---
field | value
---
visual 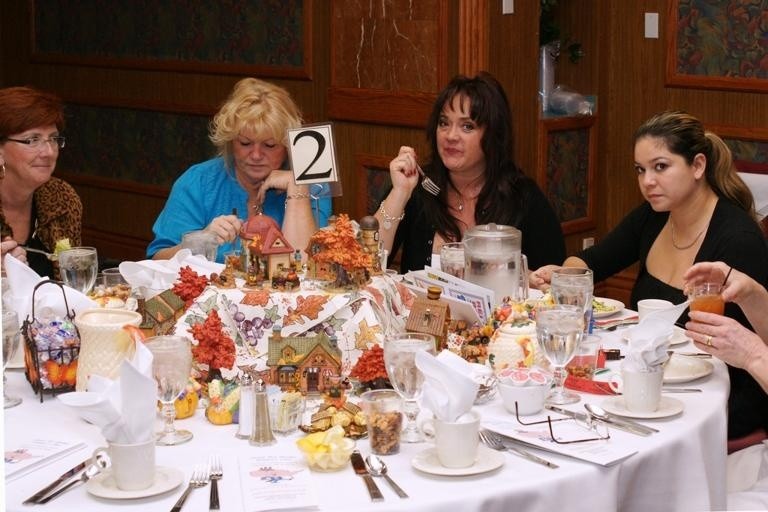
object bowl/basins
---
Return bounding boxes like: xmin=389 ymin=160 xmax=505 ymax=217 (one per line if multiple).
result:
xmin=299 ymin=438 xmax=354 ymax=473
xmin=497 ymin=382 xmax=550 ymax=415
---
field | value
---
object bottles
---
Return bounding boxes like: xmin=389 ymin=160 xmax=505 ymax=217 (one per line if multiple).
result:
xmin=235 ymin=374 xmax=254 ymax=440
xmin=251 ymin=380 xmax=278 ymax=444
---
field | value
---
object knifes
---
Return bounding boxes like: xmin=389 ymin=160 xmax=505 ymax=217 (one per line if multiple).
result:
xmin=22 ymin=458 xmax=95 ymax=505
xmin=352 ymin=450 xmax=386 ymax=503
xmin=542 ymin=402 xmax=646 ymax=442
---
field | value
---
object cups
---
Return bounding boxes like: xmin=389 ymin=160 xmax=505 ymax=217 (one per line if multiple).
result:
xmin=92 ymin=435 xmax=156 ymax=490
xmin=539 ymin=46 xmax=553 ymax=112
xmin=104 ymin=268 xmax=126 ymax=287
xmin=86 ymin=466 xmax=182 ymax=500
xmin=609 ymin=366 xmax=663 ymax=412
xmin=415 ymin=413 xmax=480 ymax=467
xmin=637 ymin=299 xmax=674 ymax=338
xmin=549 ymin=87 xmax=584 ymax=119
xmin=183 ymin=231 xmax=217 ymax=263
xmin=361 ymin=389 xmax=405 ymax=456
xmin=686 ymin=282 xmax=726 ymax=333
xmin=565 ymin=334 xmax=602 ymax=382
xmin=439 ymin=243 xmax=466 ymax=281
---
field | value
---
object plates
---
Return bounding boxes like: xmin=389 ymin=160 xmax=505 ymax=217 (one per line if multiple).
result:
xmin=609 ymin=352 xmax=712 ymax=382
xmin=411 ymin=445 xmax=508 ymax=477
xmin=594 ymin=296 xmax=626 ymax=317
xmin=620 ymin=325 xmax=690 ymax=347
xmin=603 ymin=396 xmax=683 ymax=420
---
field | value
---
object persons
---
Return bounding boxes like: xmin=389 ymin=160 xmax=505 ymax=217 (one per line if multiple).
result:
xmin=683 ymin=259 xmax=768 ymax=511
xmin=146 ymin=78 xmax=333 ymax=264
xmin=527 ymin=111 xmax=768 ymax=440
xmin=372 ymin=75 xmax=566 ymax=289
xmin=0 ymin=87 xmax=83 ymax=282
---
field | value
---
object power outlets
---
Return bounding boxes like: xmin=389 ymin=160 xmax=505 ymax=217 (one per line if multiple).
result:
xmin=583 ymin=237 xmax=595 ymax=252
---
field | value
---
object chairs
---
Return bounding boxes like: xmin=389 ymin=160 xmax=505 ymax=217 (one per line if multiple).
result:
xmin=729 ymin=169 xmax=767 ymax=460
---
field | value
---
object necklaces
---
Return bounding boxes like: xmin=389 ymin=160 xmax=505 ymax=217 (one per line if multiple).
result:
xmin=248 ymin=199 xmax=265 ymax=216
xmin=669 ymin=213 xmax=714 ymax=250
xmin=445 ymin=180 xmax=481 ymax=212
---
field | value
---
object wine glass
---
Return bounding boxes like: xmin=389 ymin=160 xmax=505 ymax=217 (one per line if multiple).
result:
xmin=384 ymin=333 xmax=436 ymax=444
xmin=551 ymin=268 xmax=594 ymax=345
xmin=2 ymin=310 xmax=24 ymax=409
xmin=537 ymin=304 xmax=584 ymax=404
xmin=141 ymin=336 xmax=194 ymax=447
xmin=58 ymin=247 xmax=98 ymax=297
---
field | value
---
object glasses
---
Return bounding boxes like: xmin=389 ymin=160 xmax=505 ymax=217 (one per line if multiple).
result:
xmin=6 ymin=136 xmax=66 ymax=150
xmin=515 ymin=401 xmax=610 ymax=444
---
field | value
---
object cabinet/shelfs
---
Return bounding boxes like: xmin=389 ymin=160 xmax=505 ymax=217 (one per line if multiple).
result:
xmin=1 ymin=2 xmax=318 ymax=271
xmin=316 ymin=0 xmax=609 ymax=262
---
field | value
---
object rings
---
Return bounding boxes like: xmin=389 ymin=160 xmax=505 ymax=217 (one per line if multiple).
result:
xmin=260 ymin=179 xmax=265 ymax=186
xmin=706 ymin=336 xmax=712 ymax=346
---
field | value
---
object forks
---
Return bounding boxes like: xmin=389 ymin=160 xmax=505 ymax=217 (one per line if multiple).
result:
xmin=596 ymin=323 xmax=640 ymax=334
xmin=414 ymin=163 xmax=440 ymax=196
xmin=170 ymin=470 xmax=208 ymax=512
xmin=17 ymin=246 xmax=59 ymax=262
xmin=209 ymin=456 xmax=224 ymax=510
xmin=478 ymin=433 xmax=560 ymax=471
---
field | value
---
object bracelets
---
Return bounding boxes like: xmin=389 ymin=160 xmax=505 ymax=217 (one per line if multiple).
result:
xmin=719 ymin=267 xmax=735 ymax=295
xmin=379 ymin=199 xmax=405 ymax=229
xmin=284 ymin=193 xmax=311 ymax=212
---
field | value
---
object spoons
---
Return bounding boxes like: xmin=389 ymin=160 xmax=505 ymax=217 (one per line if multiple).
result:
xmin=39 ymin=462 xmax=104 ymax=505
xmin=584 ymin=404 xmax=661 ymax=436
xmin=366 ymin=456 xmax=410 ymax=502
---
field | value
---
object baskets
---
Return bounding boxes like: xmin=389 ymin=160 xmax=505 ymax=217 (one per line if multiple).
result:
xmin=20 ymin=280 xmax=80 ymax=402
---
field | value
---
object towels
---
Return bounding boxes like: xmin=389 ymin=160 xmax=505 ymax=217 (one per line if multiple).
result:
xmin=414 ymin=347 xmax=495 ymax=423
xmin=118 ymin=248 xmax=227 ymax=301
xmin=619 ymin=295 xmax=695 ymax=370
xmin=3 ymin=251 xmax=91 ymax=318
xmin=50 ymin=337 xmax=165 ymax=447
